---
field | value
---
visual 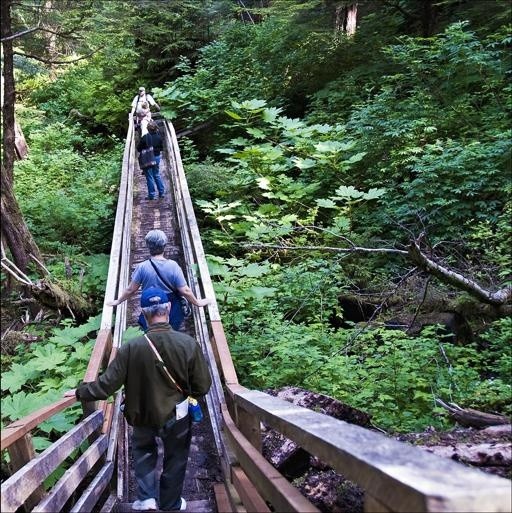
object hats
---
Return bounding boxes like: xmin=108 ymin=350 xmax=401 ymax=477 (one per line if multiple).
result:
xmin=141 ymin=287 xmax=169 ymax=307
xmin=139 ymin=86 xmax=146 ymax=90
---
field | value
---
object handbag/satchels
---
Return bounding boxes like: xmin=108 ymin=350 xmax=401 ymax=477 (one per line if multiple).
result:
xmin=187 ymin=396 xmax=204 ymax=422
xmin=179 ymin=295 xmax=192 ymax=316
xmin=138 ymin=144 xmax=157 ymax=169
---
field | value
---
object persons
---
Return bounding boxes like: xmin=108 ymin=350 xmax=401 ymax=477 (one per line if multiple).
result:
xmin=136 ymin=101 xmax=151 ymax=141
xmin=105 ymin=228 xmax=213 ymax=331
xmin=137 ymin=121 xmax=166 ymax=199
xmin=64 ymin=283 xmax=212 ymax=513
xmin=140 ymin=108 xmax=158 ymax=174
xmin=126 ymin=86 xmax=161 ymax=118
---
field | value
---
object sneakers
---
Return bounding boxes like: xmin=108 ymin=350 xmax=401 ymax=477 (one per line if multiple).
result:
xmin=180 ymin=495 xmax=187 ymax=511
xmin=145 ymin=195 xmax=155 ymax=199
xmin=132 ymin=497 xmax=158 ymax=511
xmin=159 ymin=193 xmax=165 ymax=197
